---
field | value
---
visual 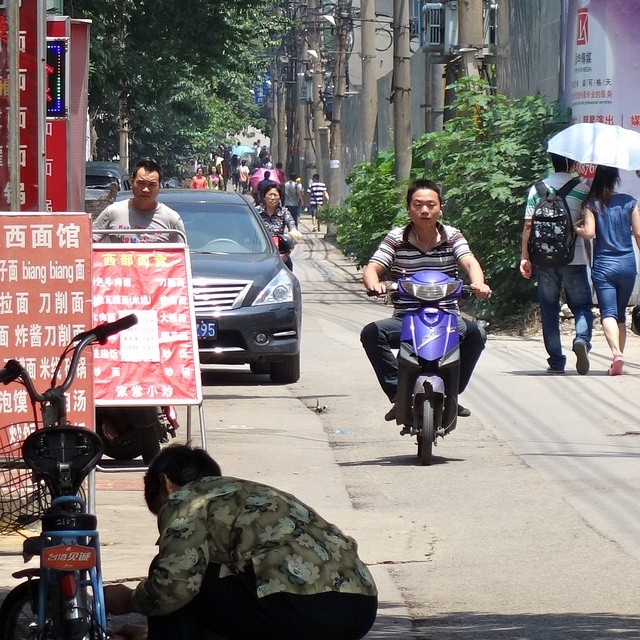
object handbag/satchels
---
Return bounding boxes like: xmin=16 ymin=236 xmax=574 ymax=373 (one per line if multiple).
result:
xmin=631 ymin=291 xmax=640 ymax=336
xmin=218 ymin=173 xmax=227 ymax=192
xmin=295 ymin=183 xmax=305 ymax=206
xmin=311 ymin=186 xmax=324 ymax=214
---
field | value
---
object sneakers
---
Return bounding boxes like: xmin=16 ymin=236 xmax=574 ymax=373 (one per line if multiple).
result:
xmin=573 ymin=338 xmax=591 ymax=375
xmin=313 ymin=227 xmax=318 ymax=233
xmin=547 ymin=368 xmax=565 ymax=374
xmin=319 ymin=228 xmax=322 ymax=232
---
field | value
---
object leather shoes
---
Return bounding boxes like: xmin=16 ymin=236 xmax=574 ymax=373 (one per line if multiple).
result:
xmin=458 ymin=404 xmax=472 ymax=417
xmin=386 ymin=402 xmax=397 ymax=423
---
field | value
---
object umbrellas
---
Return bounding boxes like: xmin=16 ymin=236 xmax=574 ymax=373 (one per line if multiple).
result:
xmin=544 ymin=123 xmax=640 ymax=171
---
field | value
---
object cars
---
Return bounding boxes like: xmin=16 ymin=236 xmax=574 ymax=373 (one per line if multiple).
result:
xmin=115 ymin=190 xmax=300 ymax=384
xmin=86 ymin=162 xmax=130 ymax=191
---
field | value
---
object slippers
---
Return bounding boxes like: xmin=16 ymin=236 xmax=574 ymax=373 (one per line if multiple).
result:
xmin=609 ymin=357 xmax=626 ymax=376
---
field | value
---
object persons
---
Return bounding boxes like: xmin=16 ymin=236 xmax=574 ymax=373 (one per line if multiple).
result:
xmin=103 ymin=442 xmax=378 ymax=640
xmin=305 ymin=173 xmax=329 ymax=232
xmin=254 ymin=184 xmax=297 ymax=236
xmin=359 ymin=178 xmax=490 ymax=421
xmin=92 ymin=161 xmax=187 ymax=244
xmin=520 ymin=154 xmax=591 ymax=375
xmin=284 ymin=174 xmax=304 ymax=229
xmin=572 ymin=165 xmax=640 ymax=375
xmin=189 ymin=140 xmax=286 ymax=190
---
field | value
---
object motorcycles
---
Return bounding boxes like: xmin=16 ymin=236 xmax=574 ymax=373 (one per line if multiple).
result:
xmin=95 ymin=406 xmax=179 ymax=466
xmin=0 ymin=314 xmax=139 ymax=640
xmin=367 ymin=271 xmax=491 ymax=462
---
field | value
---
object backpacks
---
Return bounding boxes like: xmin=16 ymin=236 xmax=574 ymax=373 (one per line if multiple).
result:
xmin=528 ymin=177 xmax=583 ymax=269
